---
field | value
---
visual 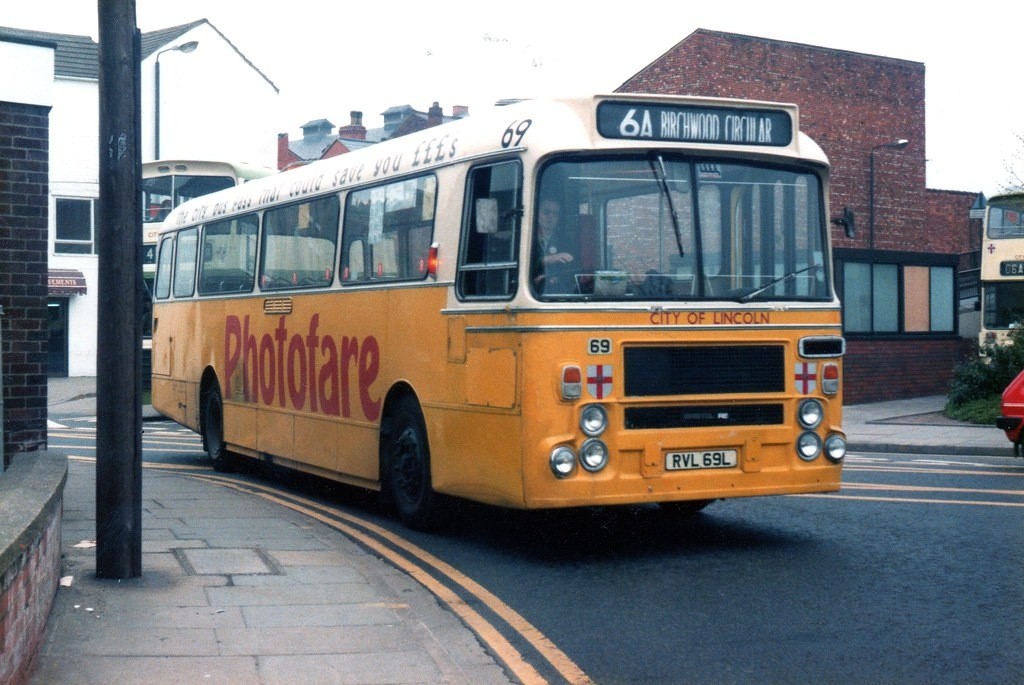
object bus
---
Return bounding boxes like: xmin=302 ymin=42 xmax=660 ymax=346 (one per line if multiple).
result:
xmin=141 ymin=160 xmax=282 ymax=390
xmin=150 ymin=92 xmax=849 ymax=528
xmin=974 ymin=191 xmax=1024 ymax=364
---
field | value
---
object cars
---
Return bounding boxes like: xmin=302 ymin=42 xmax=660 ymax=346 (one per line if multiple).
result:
xmin=994 ymin=368 xmax=1024 ymax=458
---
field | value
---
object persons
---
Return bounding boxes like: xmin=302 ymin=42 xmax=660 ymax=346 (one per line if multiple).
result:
xmin=150 ymin=199 xmax=171 ymax=222
xmin=521 ymin=198 xmax=579 ymax=296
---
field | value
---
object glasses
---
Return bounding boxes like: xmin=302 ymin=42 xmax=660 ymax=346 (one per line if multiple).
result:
xmin=541 ymin=208 xmax=559 ymax=216
